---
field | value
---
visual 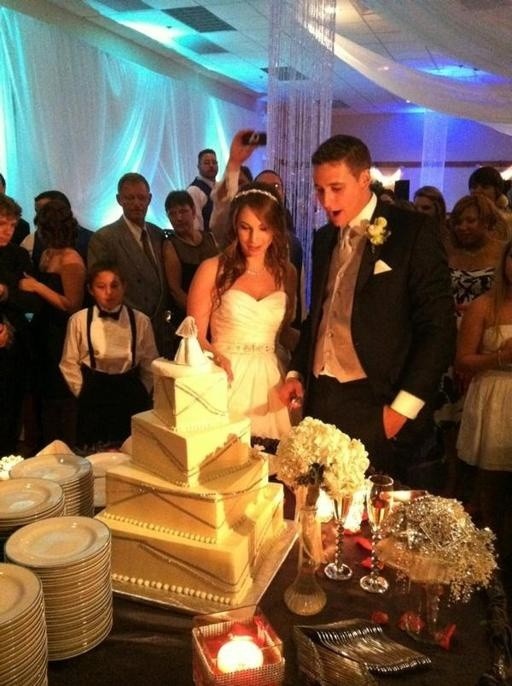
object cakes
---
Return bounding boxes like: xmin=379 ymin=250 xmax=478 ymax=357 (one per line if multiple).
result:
xmin=92 ymin=315 xmax=286 ymax=606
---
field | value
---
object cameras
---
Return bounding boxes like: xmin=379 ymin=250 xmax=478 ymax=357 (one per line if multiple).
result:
xmin=241 ymin=130 xmax=268 ymax=146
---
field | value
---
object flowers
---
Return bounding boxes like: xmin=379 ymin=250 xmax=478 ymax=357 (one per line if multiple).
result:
xmin=275 ymin=415 xmax=371 ymax=563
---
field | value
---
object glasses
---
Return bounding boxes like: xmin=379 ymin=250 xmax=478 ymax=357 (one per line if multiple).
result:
xmin=271 ymin=183 xmax=283 ymax=190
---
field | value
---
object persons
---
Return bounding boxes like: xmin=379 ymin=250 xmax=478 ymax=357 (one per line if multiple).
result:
xmin=0 ymin=127 xmax=510 ymax=534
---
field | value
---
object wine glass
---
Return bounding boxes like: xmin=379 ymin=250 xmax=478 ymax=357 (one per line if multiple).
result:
xmin=324 ymin=492 xmax=352 ymax=581
xmin=361 ymin=474 xmax=393 ymax=594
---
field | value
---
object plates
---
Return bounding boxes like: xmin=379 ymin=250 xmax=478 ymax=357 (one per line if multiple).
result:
xmin=1 ymin=452 xmax=134 ymax=685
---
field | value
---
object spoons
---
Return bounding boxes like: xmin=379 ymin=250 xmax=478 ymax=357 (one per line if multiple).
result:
xmin=309 ymin=619 xmax=433 ymax=677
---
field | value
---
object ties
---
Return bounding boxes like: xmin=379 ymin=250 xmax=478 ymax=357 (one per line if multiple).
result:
xmin=140 ymin=229 xmax=160 ymax=277
xmin=336 ymin=226 xmax=355 ymax=267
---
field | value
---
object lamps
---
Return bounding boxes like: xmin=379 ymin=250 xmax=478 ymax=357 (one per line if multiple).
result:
xmin=191 ymin=604 xmax=286 ymax=685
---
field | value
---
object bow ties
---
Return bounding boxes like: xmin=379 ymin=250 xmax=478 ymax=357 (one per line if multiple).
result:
xmin=99 ymin=310 xmax=120 ymax=321
